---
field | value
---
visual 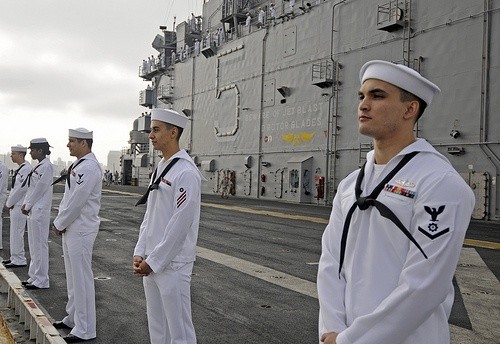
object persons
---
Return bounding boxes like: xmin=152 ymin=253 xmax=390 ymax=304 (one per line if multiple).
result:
xmin=142 ymin=0 xmax=312 ymax=75
xmin=134 ymin=107 xmax=199 ymax=343
xmin=104 ymin=170 xmax=123 ymax=191
xmin=49 ymin=127 xmax=103 ymax=343
xmin=5 ymin=144 xmax=27 ymax=268
xmin=314 ymin=58 xmax=475 ymax=342
xmin=142 ymin=84 xmax=152 ymax=117
xmin=21 ymin=139 xmax=49 ymax=291
xmin=219 ymin=175 xmax=232 ymax=200
xmin=0 ymin=161 xmax=10 ymax=251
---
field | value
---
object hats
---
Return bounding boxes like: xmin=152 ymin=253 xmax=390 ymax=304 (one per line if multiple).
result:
xmin=11 ymin=145 xmax=28 ymax=152
xmin=151 ymin=108 xmax=188 ymax=129
xmin=359 ymin=60 xmax=441 ymax=107
xmin=28 ymin=138 xmax=51 ymax=148
xmin=69 ymin=128 xmax=93 ymax=139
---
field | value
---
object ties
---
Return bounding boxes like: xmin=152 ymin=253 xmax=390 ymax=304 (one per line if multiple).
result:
xmin=11 ymin=163 xmax=25 ymax=188
xmin=134 ymin=158 xmax=180 ymax=206
xmin=338 ymin=152 xmax=427 ymax=279
xmin=21 ymin=163 xmax=41 ymax=188
xmin=51 ymin=159 xmax=86 ymax=189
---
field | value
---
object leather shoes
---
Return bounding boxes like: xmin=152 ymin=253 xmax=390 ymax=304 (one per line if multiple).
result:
xmin=3 ymin=259 xmax=96 ymax=343
